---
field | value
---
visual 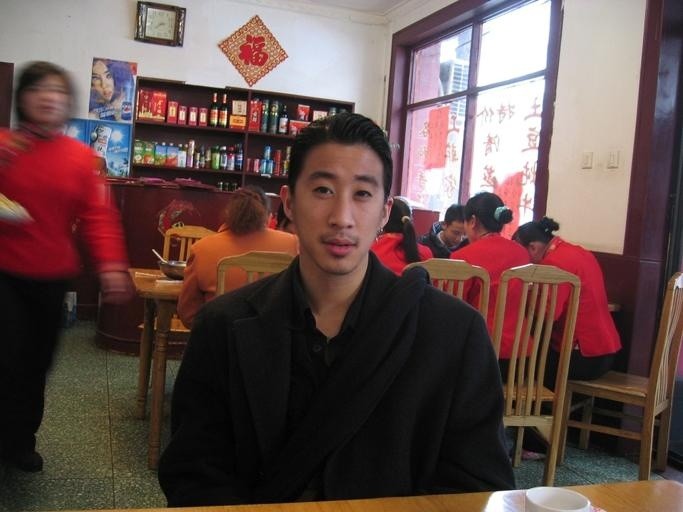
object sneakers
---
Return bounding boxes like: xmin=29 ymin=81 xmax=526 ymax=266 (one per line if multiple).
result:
xmin=509 ymin=444 xmax=548 ymax=462
xmin=1 ymin=443 xmax=44 ymax=473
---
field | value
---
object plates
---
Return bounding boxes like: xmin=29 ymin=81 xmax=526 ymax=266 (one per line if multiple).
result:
xmin=484 ymin=489 xmax=607 ymax=511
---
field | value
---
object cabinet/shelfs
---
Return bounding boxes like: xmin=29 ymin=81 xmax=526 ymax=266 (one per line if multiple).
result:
xmin=129 ymin=75 xmax=356 ymax=191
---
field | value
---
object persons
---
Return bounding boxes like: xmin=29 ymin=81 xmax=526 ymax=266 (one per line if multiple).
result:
xmin=0 ymin=62 xmax=136 ymax=472
xmin=90 ymin=58 xmax=134 ymax=123
xmin=157 ymin=111 xmax=518 ymax=506
xmin=178 ymin=187 xmax=298 ymax=329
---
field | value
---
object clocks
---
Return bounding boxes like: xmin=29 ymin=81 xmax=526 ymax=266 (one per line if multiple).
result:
xmin=134 ymin=0 xmax=186 ymax=47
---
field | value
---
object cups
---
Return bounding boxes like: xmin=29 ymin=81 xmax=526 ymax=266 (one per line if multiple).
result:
xmin=216 ymin=181 xmax=238 ymax=192
xmin=524 ymin=485 xmax=593 ymax=511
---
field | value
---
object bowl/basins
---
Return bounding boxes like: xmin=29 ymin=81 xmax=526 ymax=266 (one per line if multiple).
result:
xmin=157 ymin=261 xmax=185 ymax=280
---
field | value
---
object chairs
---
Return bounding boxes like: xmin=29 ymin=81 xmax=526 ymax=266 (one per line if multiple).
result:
xmin=552 ymin=270 xmax=683 ymax=481
xmin=160 ymin=226 xmax=217 ymax=261
xmin=400 ymin=258 xmax=490 ymax=325
xmin=213 ymin=250 xmax=295 ymax=297
xmin=490 ymin=262 xmax=582 ymax=487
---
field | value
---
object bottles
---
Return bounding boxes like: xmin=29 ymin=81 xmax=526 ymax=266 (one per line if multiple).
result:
xmin=219 ymin=94 xmax=230 ymax=126
xmin=209 ymin=93 xmax=218 ymax=127
xmin=328 ymin=106 xmax=346 ymax=117
xmin=277 ymin=104 xmax=289 ymax=135
xmin=177 ymin=139 xmax=242 ymax=171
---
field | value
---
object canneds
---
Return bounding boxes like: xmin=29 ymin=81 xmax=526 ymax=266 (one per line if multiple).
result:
xmin=246 ymin=144 xmax=292 ymax=176
xmin=186 ymin=140 xmax=200 ymax=169
xmin=121 ymin=102 xmax=131 ymax=120
